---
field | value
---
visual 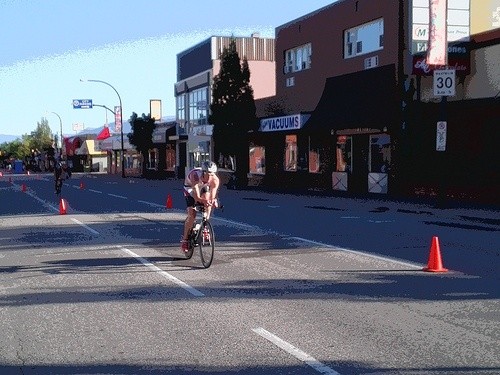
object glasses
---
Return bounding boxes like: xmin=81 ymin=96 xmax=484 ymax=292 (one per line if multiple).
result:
xmin=204 ymin=172 xmax=215 ymax=176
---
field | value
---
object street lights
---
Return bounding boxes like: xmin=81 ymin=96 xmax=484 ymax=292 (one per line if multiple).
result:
xmin=80 ymin=78 xmax=126 ymax=179
xmin=44 ymin=110 xmax=63 ymax=160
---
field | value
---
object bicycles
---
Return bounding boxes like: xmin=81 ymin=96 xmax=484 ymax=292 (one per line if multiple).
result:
xmin=53 ymin=177 xmax=63 ymax=197
xmin=179 ymin=201 xmax=224 ymax=268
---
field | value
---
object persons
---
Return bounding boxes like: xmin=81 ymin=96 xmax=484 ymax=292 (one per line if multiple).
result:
xmin=181 ymin=160 xmax=220 ymax=253
xmin=54 ymin=163 xmax=65 ymax=194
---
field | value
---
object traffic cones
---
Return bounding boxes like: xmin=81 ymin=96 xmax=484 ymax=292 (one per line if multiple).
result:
xmin=165 ymin=193 xmax=173 ymax=209
xmin=423 ymin=237 xmax=448 ymax=272
xmin=80 ymin=182 xmax=85 ymax=191
xmin=60 ymin=198 xmax=67 ymax=214
xmin=22 ymin=183 xmax=26 ymax=191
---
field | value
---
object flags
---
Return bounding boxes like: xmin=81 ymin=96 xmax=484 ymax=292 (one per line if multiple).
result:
xmin=95 ymin=127 xmax=110 ymax=140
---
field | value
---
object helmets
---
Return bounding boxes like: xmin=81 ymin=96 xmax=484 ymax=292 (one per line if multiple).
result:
xmin=202 ymin=161 xmax=217 ymax=172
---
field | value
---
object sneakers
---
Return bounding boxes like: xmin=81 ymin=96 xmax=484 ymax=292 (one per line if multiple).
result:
xmin=204 ymin=230 xmax=209 ymax=240
xmin=182 ymin=239 xmax=189 ymax=253
